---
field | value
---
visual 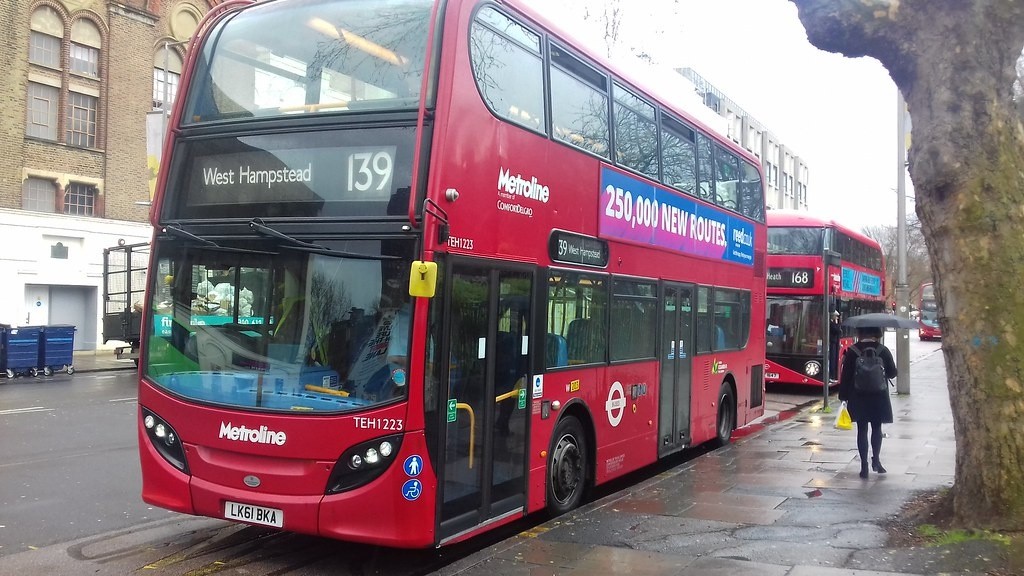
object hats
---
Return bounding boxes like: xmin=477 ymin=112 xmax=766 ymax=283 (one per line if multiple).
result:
xmin=830 ymin=310 xmax=840 ymax=316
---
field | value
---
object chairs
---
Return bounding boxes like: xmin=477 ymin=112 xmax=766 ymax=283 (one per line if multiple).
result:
xmin=325 ymin=313 xmax=391 ymax=400
xmin=470 ymin=310 xmax=729 ymax=385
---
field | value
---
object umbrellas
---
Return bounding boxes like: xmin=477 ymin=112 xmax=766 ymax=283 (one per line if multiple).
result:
xmin=842 ymin=313 xmax=923 ymax=346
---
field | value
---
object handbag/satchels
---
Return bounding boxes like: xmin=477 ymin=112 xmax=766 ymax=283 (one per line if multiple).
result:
xmin=833 ymin=400 xmax=853 ymax=430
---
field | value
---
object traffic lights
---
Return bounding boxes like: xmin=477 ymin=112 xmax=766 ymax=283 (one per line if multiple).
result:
xmin=892 ymin=302 xmax=896 ymax=309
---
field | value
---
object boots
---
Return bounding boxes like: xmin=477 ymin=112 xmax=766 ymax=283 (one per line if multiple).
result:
xmin=871 ymin=456 xmax=886 ymax=474
xmin=859 ymin=462 xmax=868 ymax=480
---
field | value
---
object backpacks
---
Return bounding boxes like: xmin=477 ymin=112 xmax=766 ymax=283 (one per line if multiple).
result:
xmin=847 ymin=344 xmax=889 ymax=399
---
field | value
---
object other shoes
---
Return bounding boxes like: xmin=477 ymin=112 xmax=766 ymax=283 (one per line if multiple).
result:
xmin=828 ymin=381 xmax=832 ymax=385
xmin=830 ymin=379 xmax=838 ymax=383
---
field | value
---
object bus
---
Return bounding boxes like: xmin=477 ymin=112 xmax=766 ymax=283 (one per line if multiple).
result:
xmin=919 ymin=283 xmax=942 ymax=342
xmin=764 ymin=215 xmax=885 ymax=390
xmin=137 ymin=1 xmax=767 ymax=550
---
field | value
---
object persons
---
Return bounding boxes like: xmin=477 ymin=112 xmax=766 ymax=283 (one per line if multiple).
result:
xmin=279 ymin=254 xmax=332 ymax=321
xmin=387 ymin=278 xmax=437 ymax=469
xmin=830 ymin=310 xmax=841 ymax=383
xmin=837 ymin=327 xmax=898 ymax=479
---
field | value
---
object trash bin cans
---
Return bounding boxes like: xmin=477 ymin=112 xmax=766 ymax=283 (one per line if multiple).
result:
xmin=0 ymin=324 xmax=44 ymax=379
xmin=38 ymin=324 xmax=77 ymax=377
